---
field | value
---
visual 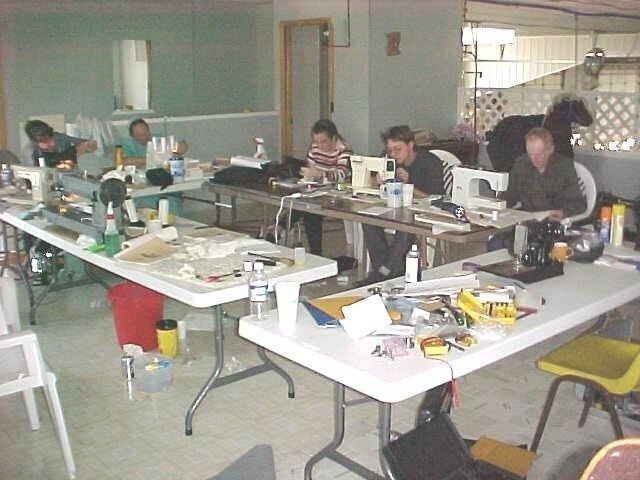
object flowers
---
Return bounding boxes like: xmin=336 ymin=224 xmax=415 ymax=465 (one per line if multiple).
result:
xmin=447 ymin=122 xmax=483 ymax=143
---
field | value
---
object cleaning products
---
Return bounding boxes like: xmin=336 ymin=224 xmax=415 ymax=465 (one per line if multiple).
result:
xmin=103 ymin=200 xmax=121 ymax=257
xmin=251 ymin=137 xmax=268 ymax=161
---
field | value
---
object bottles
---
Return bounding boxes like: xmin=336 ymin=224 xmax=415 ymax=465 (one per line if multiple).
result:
xmin=403 ymin=244 xmax=424 ymax=284
xmin=0 ymin=164 xmax=27 ymax=194
xmin=102 ymin=201 xmax=120 ymax=255
xmin=293 ymin=241 xmax=306 ymax=265
xmin=598 ymin=202 xmax=627 ymax=251
xmin=168 ymin=150 xmax=185 ymax=181
xmin=156 ymin=319 xmax=178 ymax=358
xmin=247 ymin=261 xmax=270 ymax=323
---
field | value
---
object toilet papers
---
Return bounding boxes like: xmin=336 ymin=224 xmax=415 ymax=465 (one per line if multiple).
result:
xmin=121 ymin=226 xmax=178 ymax=250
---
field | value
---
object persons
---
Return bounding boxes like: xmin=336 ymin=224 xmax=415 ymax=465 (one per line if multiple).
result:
xmin=23 ymin=120 xmax=98 ymax=254
xmin=266 ymin=119 xmax=353 ymax=256
xmin=120 ymin=119 xmax=188 ymax=217
xmin=351 ymin=126 xmax=445 ymax=288
xmin=487 ymin=128 xmax=587 ymax=251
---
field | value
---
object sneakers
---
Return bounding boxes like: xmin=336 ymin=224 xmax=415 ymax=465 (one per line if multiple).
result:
xmin=263 ymin=222 xmax=284 ymax=243
xmin=351 ymin=275 xmax=387 ymax=289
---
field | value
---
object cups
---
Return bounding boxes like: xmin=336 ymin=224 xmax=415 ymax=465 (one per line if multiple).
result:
xmin=379 ymin=178 xmax=415 ymax=211
xmin=120 ymin=351 xmax=134 ymax=380
xmin=514 ymin=284 xmax=544 ymax=314
xmin=515 ymin=240 xmax=575 ymax=268
xmin=273 ymin=282 xmax=302 ymax=324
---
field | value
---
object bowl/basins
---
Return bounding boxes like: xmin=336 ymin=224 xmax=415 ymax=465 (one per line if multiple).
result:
xmin=132 ymin=354 xmax=176 ymax=394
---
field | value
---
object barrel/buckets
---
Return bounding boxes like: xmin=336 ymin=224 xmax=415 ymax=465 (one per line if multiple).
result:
xmin=104 ymin=284 xmax=166 ymax=352
